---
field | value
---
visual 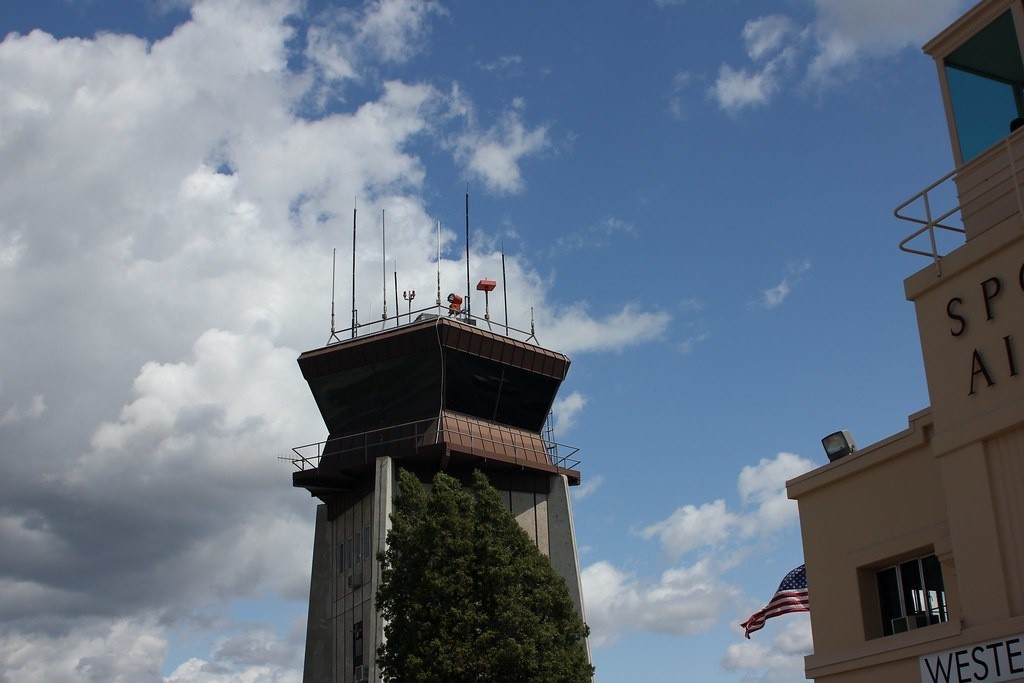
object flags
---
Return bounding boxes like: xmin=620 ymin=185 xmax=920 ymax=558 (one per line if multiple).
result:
xmin=739 ymin=563 xmax=812 ymax=640
